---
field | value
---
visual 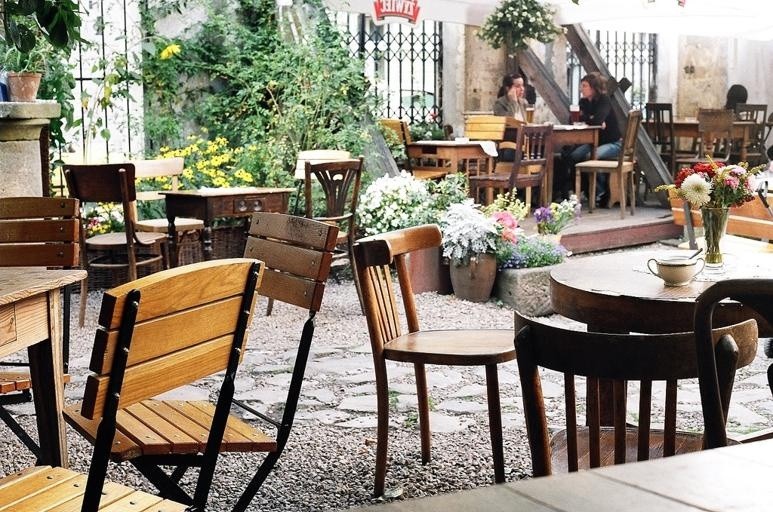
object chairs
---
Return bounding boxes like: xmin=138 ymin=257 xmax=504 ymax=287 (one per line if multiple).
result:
xmin=352 ymin=224 xmax=517 ymax=497
xmin=512 ymin=309 xmax=758 ymax=477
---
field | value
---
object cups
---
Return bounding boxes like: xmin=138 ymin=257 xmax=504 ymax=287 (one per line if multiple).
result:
xmin=647 ymin=258 xmax=705 ymax=287
xmin=570 ymin=105 xmax=581 ymax=125
xmin=525 ymin=103 xmax=536 ymax=123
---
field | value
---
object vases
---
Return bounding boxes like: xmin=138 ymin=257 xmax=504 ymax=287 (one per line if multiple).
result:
xmin=395 ymin=246 xmax=449 ymax=295
xmin=699 ymin=209 xmax=728 ymax=272
xmin=446 ymin=251 xmax=499 ymax=303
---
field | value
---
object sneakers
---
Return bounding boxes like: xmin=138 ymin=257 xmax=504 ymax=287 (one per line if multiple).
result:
xmin=598 ymin=191 xmax=610 ymax=209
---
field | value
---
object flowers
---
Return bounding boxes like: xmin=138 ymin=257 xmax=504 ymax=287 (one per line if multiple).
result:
xmin=654 ymin=152 xmax=766 ymax=263
xmin=475 ymin=1 xmax=568 ymax=56
xmin=357 ymin=175 xmax=582 ymax=265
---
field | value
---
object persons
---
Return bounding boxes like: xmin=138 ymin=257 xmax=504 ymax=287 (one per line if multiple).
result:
xmin=492 ymin=71 xmax=529 ymax=163
xmin=559 ymin=72 xmax=622 ymax=204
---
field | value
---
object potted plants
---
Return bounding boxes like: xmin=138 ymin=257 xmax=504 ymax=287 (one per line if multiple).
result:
xmin=0 ymin=15 xmax=54 ymax=101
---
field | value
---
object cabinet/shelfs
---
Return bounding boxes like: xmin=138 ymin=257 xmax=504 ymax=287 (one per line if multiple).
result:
xmin=694 ymin=280 xmax=773 ymax=447
